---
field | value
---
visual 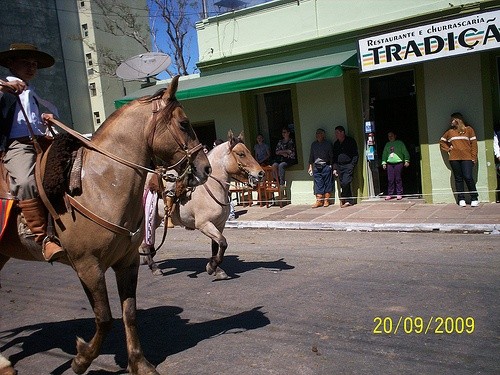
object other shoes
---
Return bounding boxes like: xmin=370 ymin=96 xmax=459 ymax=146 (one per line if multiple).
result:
xmin=385 ymin=197 xmax=391 ymax=200
xmin=396 ymin=195 xmax=402 ymax=199
xmin=276 ymin=185 xmax=283 ymax=188
xmin=341 ymin=202 xmax=352 ymax=207
xmin=271 ymin=184 xmax=275 ymax=188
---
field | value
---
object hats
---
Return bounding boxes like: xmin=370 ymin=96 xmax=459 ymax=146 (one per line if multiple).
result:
xmin=0 ymin=43 xmax=55 ymax=69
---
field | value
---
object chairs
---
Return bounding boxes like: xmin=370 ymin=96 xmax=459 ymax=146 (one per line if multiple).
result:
xmin=230 ymin=166 xmax=288 ymax=208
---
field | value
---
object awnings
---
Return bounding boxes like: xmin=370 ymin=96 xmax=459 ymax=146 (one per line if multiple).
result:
xmin=115 ymin=49 xmax=358 ymax=109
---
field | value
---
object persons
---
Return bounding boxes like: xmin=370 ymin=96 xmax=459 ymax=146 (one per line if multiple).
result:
xmin=439 ymin=112 xmax=479 ymax=207
xmin=307 ymin=128 xmax=334 ymax=208
xmin=0 ymin=43 xmax=66 ymax=263
xmin=493 ymin=129 xmax=500 ymax=202
xmin=203 ymin=139 xmax=236 ymax=221
xmin=271 ymin=127 xmax=297 ymax=187
xmin=331 ymin=126 xmax=359 ymax=208
xmin=252 ymin=133 xmax=270 ymax=167
xmin=381 ymin=132 xmax=410 ymax=200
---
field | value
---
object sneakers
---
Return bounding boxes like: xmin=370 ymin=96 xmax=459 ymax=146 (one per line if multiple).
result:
xmin=459 ymin=200 xmax=466 ymax=206
xmin=471 ymin=200 xmax=479 ymax=207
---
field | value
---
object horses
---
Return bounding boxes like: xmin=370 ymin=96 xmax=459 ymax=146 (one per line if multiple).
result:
xmin=2 ymin=73 xmax=212 ymax=375
xmin=140 ymin=129 xmax=267 ymax=278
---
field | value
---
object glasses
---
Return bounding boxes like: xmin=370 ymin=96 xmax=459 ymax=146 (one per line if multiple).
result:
xmin=282 ymin=132 xmax=286 ymax=133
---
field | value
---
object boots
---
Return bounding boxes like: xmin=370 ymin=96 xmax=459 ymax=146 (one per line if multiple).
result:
xmin=312 ymin=194 xmax=323 ymax=208
xmin=323 ymin=193 xmax=330 ymax=206
xmin=164 ymin=196 xmax=174 ymax=228
xmin=19 ymin=195 xmax=65 ymax=262
